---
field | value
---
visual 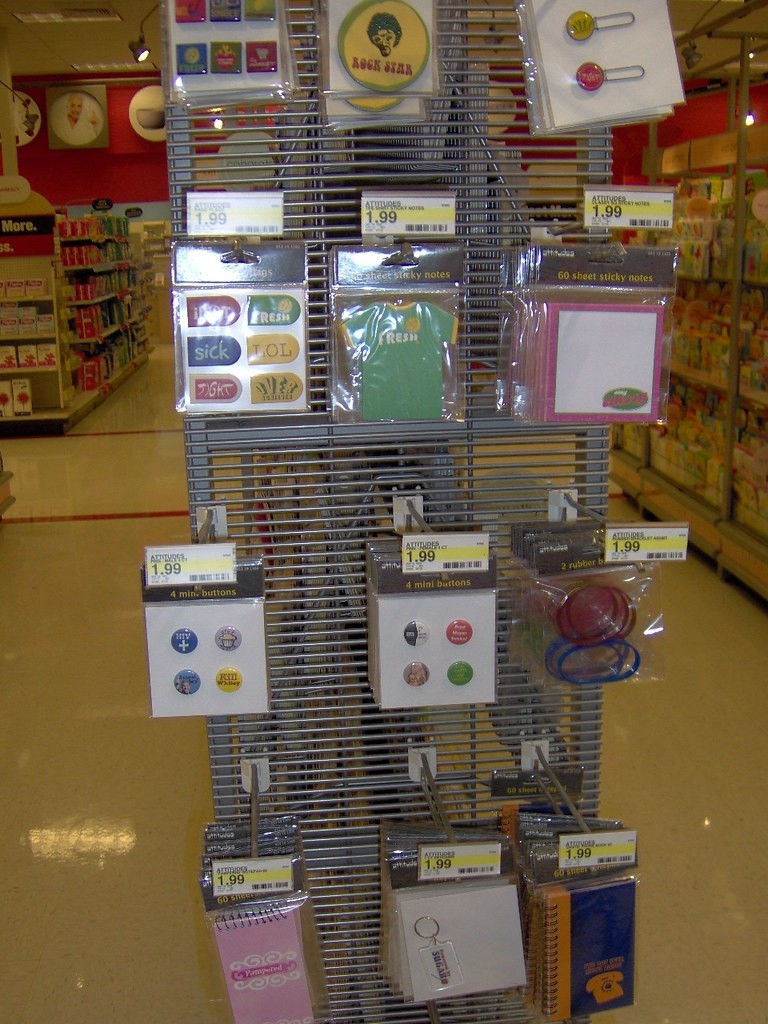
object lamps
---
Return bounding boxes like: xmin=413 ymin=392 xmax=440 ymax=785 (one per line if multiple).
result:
xmin=681 ymin=39 xmax=704 ymax=69
xmin=128 ymin=1 xmax=163 ymax=63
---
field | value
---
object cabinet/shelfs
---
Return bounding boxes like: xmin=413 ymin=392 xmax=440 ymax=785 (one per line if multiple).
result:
xmin=1 ymin=226 xmax=157 ymax=434
xmin=167 ymin=0 xmax=613 ymax=1024
xmin=606 ymin=122 xmax=768 ymax=607
